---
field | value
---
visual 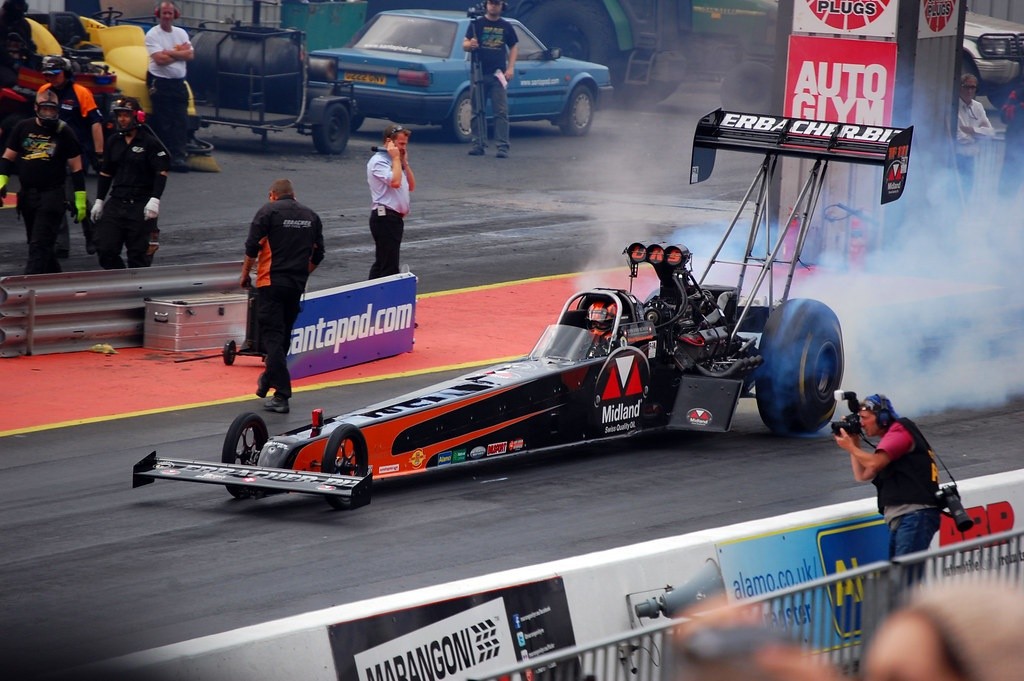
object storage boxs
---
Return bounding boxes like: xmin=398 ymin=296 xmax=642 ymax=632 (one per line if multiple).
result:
xmin=143 ymin=291 xmax=247 ymax=353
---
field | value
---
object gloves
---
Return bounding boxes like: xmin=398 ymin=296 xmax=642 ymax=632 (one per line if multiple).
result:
xmin=143 ymin=196 xmax=160 ymax=221
xmin=90 ymin=198 xmax=103 ymax=223
xmin=70 ymin=191 xmax=88 ymax=224
xmin=0 ymin=175 xmax=9 ymax=208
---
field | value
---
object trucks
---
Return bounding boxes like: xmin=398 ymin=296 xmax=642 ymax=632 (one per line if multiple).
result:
xmin=342 ymin=0 xmax=781 ymax=122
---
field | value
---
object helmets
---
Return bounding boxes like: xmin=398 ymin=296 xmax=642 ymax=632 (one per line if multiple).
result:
xmin=112 ymin=96 xmax=142 ymax=133
xmin=42 ymin=54 xmax=72 ymax=82
xmin=34 ymin=89 xmax=58 ymax=126
xmin=588 ymin=301 xmax=616 ymax=335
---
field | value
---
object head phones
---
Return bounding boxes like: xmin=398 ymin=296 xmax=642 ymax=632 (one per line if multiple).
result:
xmin=154 ymin=1 xmax=180 ymax=19
xmin=59 ymin=56 xmax=73 ymax=80
xmin=874 ymin=393 xmax=891 ymax=429
xmin=136 ymin=110 xmax=147 ymax=124
xmin=484 ymin=0 xmax=509 ymax=11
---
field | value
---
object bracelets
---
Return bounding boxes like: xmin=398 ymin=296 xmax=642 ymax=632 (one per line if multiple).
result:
xmin=403 ymin=163 xmax=408 ymax=167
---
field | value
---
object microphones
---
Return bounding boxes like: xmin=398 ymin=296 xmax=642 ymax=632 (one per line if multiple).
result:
xmin=372 ymin=146 xmax=387 ymax=152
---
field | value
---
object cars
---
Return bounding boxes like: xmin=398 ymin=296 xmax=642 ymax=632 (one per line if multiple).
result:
xmin=964 ymin=7 xmax=1024 ymax=98
xmin=310 ymin=6 xmax=615 ymax=142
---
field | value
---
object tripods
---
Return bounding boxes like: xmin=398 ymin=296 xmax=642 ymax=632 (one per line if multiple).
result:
xmin=471 ymin=18 xmax=489 ymax=149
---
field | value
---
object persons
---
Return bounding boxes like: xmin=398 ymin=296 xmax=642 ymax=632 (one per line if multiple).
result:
xmin=835 ymin=393 xmax=940 ymax=607
xmin=35 ymin=55 xmax=104 ymax=257
xmin=90 ymin=97 xmax=170 ymax=270
xmin=671 ymin=575 xmax=1024 ymax=681
xmin=584 ymin=300 xmax=617 ymax=341
xmin=954 ymin=73 xmax=996 ymax=199
xmin=0 ymin=0 xmax=42 ymax=88
xmin=0 ymin=90 xmax=87 ymax=274
xmin=462 ymin=0 xmax=519 ymax=157
xmin=1000 ymin=76 xmax=1024 ymax=197
xmin=366 ymin=125 xmax=415 ymax=279
xmin=239 ymin=178 xmax=325 ymax=414
xmin=145 ymin=0 xmax=195 ymax=173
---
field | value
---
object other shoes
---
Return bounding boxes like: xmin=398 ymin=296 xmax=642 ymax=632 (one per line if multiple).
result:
xmin=54 ymin=245 xmax=70 ymax=259
xmin=85 ymin=235 xmax=96 ymax=254
xmin=469 ymin=147 xmax=485 ymax=156
xmin=497 ymin=150 xmax=508 ymax=157
xmin=264 ymin=392 xmax=289 ymax=412
xmin=256 ymin=373 xmax=269 ymax=399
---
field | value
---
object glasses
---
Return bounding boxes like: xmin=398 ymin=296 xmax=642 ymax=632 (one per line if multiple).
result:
xmin=387 ymin=126 xmax=403 ymax=138
xmin=962 ymin=84 xmax=976 ymax=90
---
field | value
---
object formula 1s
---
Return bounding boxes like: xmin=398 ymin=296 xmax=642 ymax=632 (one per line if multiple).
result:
xmin=129 ymin=105 xmax=916 ymax=514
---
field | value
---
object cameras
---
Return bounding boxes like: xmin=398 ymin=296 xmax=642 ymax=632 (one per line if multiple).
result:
xmin=935 ymin=484 xmax=974 ymax=532
xmin=831 ymin=389 xmax=863 ymax=435
xmin=466 ymin=2 xmax=488 ymax=17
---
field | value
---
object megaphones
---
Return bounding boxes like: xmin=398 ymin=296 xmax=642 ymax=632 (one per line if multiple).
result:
xmin=635 ymin=557 xmax=728 ymax=620
